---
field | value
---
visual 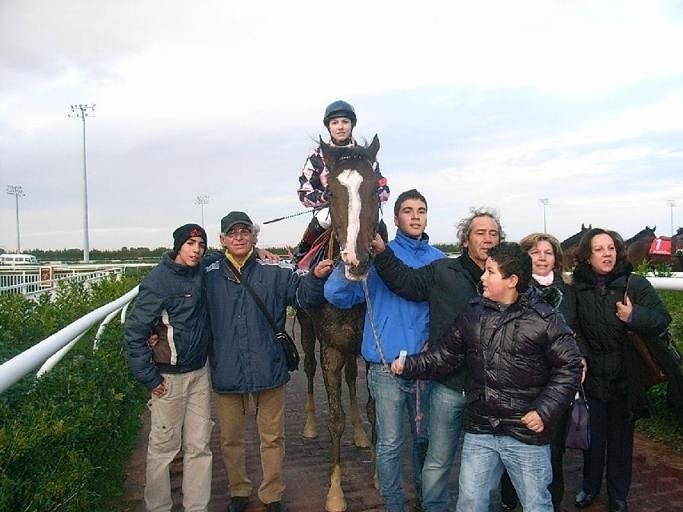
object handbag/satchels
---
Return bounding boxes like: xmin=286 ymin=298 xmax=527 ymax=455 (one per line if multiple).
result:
xmin=622 ymin=273 xmax=681 ymax=389
xmin=275 ymin=331 xmax=300 ymax=372
xmin=564 ymin=382 xmax=591 ymax=450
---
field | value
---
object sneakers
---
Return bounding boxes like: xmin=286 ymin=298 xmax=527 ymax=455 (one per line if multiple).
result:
xmin=609 ymin=498 xmax=626 ymax=511
xmin=500 ymin=498 xmax=517 ymax=510
xmin=264 ymin=500 xmax=288 ymax=512
xmin=227 ymin=496 xmax=248 ymax=511
xmin=574 ymin=489 xmax=595 ymax=506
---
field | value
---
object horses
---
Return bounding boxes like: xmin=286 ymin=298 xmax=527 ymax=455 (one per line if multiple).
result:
xmin=622 ymin=225 xmax=683 ymax=271
xmin=560 ymin=224 xmax=592 ymax=269
xmin=295 ymin=133 xmax=389 ymax=512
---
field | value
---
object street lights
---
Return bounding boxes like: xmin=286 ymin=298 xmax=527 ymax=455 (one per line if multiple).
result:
xmin=538 ymin=197 xmax=549 ymax=236
xmin=664 ymin=199 xmax=676 ymax=237
xmin=194 ymin=193 xmax=209 ymax=231
xmin=63 ymin=101 xmax=95 ymax=263
xmin=6 ymin=183 xmax=24 ymax=253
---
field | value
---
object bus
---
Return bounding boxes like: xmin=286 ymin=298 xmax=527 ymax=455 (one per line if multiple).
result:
xmin=0 ymin=253 xmax=38 ymax=265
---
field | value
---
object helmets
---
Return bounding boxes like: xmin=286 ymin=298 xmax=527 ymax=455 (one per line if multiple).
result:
xmin=323 ymin=100 xmax=356 ymax=128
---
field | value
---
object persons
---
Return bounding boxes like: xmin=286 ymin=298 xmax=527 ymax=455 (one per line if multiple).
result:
xmin=369 ymin=208 xmax=501 ymax=512
xmin=294 ymin=99 xmax=389 ymax=254
xmin=324 ymin=189 xmax=448 ymax=511
xmin=571 ymin=228 xmax=672 ymax=512
xmin=389 ymin=242 xmax=583 ymax=512
xmin=148 ymin=211 xmax=333 ymax=512
xmin=124 ymin=225 xmax=281 ymax=512
xmin=502 ymin=233 xmax=588 ymax=511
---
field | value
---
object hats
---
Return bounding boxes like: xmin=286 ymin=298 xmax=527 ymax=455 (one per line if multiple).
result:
xmin=220 ymin=211 xmax=253 ymax=234
xmin=172 ymin=223 xmax=207 ymax=253
